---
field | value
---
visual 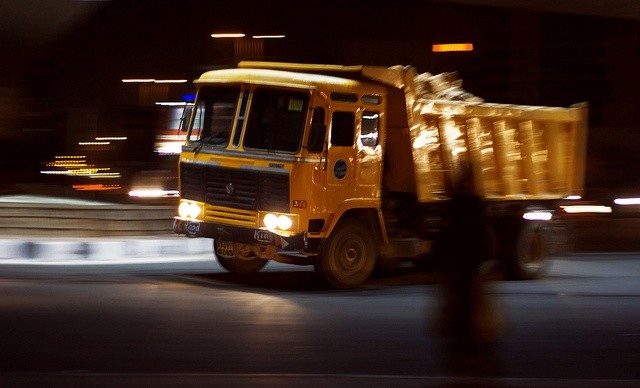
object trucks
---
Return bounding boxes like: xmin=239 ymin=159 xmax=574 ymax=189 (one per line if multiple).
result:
xmin=173 ymin=59 xmax=587 ymax=291
